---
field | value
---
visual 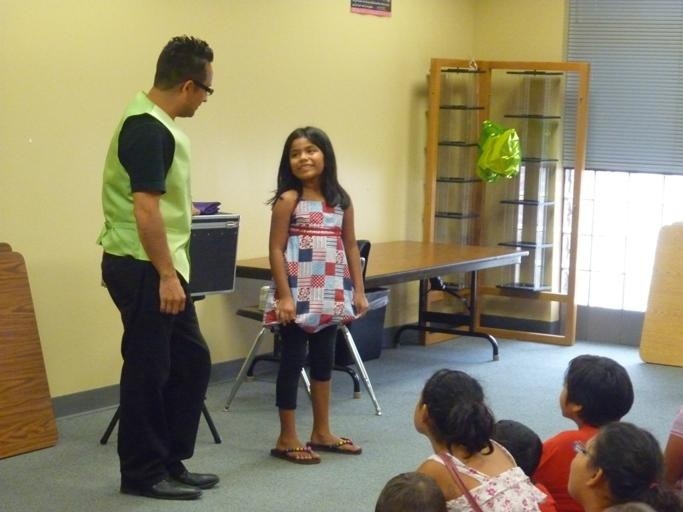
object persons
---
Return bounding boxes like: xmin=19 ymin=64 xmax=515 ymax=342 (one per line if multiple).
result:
xmin=96 ymin=35 xmax=219 ymax=500
xmin=490 ymin=420 xmax=542 ymax=484
xmin=414 ymin=368 xmax=548 ymax=512
xmin=375 ymin=472 xmax=447 ymax=512
xmin=663 ymin=410 xmax=683 ymax=490
xmin=568 ymin=422 xmax=683 ymax=512
xmin=532 ymin=354 xmax=633 ymax=512
xmin=264 ymin=127 xmax=370 ymax=464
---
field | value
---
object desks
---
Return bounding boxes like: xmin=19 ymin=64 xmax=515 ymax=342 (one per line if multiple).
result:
xmin=234 ymin=239 xmax=530 ymax=399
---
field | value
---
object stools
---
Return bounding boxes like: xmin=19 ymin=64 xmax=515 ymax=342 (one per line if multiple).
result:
xmin=99 ymin=292 xmax=222 ymax=446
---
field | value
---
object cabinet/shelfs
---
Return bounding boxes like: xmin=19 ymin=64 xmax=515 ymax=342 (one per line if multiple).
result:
xmin=420 ymin=56 xmax=591 ymax=345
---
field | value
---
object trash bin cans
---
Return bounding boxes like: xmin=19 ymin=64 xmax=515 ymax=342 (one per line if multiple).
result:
xmin=335 ymin=287 xmax=390 ymax=366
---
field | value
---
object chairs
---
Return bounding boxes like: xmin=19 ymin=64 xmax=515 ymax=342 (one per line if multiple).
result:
xmin=222 ymin=238 xmax=382 ymax=421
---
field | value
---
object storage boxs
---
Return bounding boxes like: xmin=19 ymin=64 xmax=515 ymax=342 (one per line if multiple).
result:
xmin=187 ymin=214 xmax=241 ymax=297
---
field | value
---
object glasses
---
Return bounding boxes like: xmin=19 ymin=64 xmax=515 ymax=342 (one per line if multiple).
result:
xmin=189 ymin=77 xmax=213 ymax=97
xmin=571 ymin=439 xmax=607 ymax=479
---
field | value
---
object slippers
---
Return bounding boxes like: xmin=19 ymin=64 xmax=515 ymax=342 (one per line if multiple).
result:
xmin=269 ymin=446 xmax=321 ymax=465
xmin=305 ymin=436 xmax=363 ymax=454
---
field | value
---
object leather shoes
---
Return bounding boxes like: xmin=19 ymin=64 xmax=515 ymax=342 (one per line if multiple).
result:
xmin=119 ymin=475 xmax=204 ymax=500
xmin=169 ymin=469 xmax=220 ymax=489
